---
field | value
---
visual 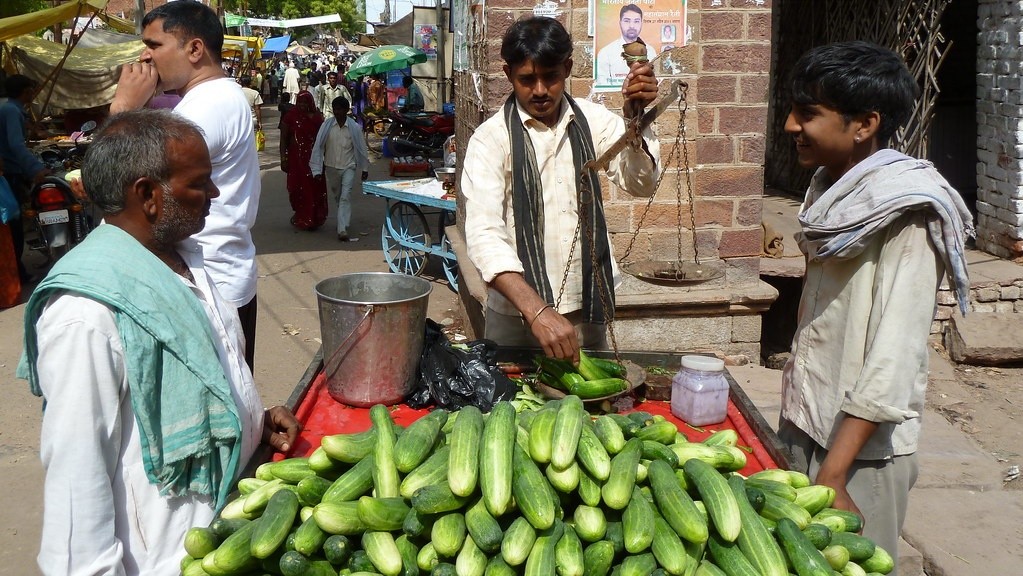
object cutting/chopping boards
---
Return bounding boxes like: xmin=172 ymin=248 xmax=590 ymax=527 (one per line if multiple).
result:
xmin=635 ymin=367 xmax=678 ymax=401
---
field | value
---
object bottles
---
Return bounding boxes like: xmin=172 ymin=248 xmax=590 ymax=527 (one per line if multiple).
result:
xmin=669 ymin=356 xmax=730 ymax=427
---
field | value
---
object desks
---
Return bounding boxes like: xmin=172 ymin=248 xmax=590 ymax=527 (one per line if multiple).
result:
xmin=236 ymin=342 xmax=803 ymax=509
xmin=361 ymin=178 xmax=460 ymax=294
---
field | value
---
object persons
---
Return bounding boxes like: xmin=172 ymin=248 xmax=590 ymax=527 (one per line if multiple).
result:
xmin=598 ymin=4 xmax=657 ymax=83
xmin=0 ymin=73 xmax=52 ymax=310
xmin=783 ymin=42 xmax=977 ymax=575
xmin=663 ymin=26 xmax=674 ymax=41
xmin=109 ymin=1 xmax=261 ymax=379
xmin=15 ymin=107 xmax=298 ymax=576
xmin=220 ymin=44 xmax=387 ymax=242
xmin=460 ymin=17 xmax=659 ymax=368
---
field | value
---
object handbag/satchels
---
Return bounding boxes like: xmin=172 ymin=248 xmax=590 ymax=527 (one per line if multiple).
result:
xmin=256 ymin=129 xmax=267 ymax=153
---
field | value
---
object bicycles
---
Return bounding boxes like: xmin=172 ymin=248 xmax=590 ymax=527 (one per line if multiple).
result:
xmin=363 ymin=115 xmax=393 ymax=155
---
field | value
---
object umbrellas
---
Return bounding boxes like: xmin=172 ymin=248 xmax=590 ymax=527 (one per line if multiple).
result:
xmin=345 ymin=45 xmax=427 ymax=92
xmin=286 ymin=44 xmax=315 ymax=55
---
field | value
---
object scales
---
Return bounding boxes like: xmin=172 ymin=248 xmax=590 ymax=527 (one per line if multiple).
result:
xmin=433 ymin=168 xmax=456 ymax=200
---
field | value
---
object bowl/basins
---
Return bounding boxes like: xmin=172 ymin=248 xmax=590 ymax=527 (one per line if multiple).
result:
xmin=433 ymin=167 xmax=456 ymax=182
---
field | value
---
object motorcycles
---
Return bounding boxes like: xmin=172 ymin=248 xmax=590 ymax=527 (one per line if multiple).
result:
xmin=26 ymin=120 xmax=96 ymax=266
xmin=387 ymin=110 xmax=456 ymax=158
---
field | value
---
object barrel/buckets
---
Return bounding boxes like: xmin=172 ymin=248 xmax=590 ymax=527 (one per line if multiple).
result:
xmin=316 ymin=271 xmax=431 ymax=407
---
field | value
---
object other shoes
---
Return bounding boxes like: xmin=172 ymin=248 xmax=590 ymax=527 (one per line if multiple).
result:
xmin=290 ymin=214 xmax=296 ymax=226
xmin=22 ymin=274 xmax=36 ymax=285
xmin=337 ymin=231 xmax=350 ymax=241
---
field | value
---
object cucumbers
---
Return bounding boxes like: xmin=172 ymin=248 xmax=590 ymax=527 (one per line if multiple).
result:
xmin=180 ymin=395 xmax=895 ymax=576
xmin=508 ymin=347 xmax=627 ymax=408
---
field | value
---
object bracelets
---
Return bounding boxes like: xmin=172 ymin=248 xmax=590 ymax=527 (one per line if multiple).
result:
xmin=528 ymin=303 xmax=555 ymax=327
